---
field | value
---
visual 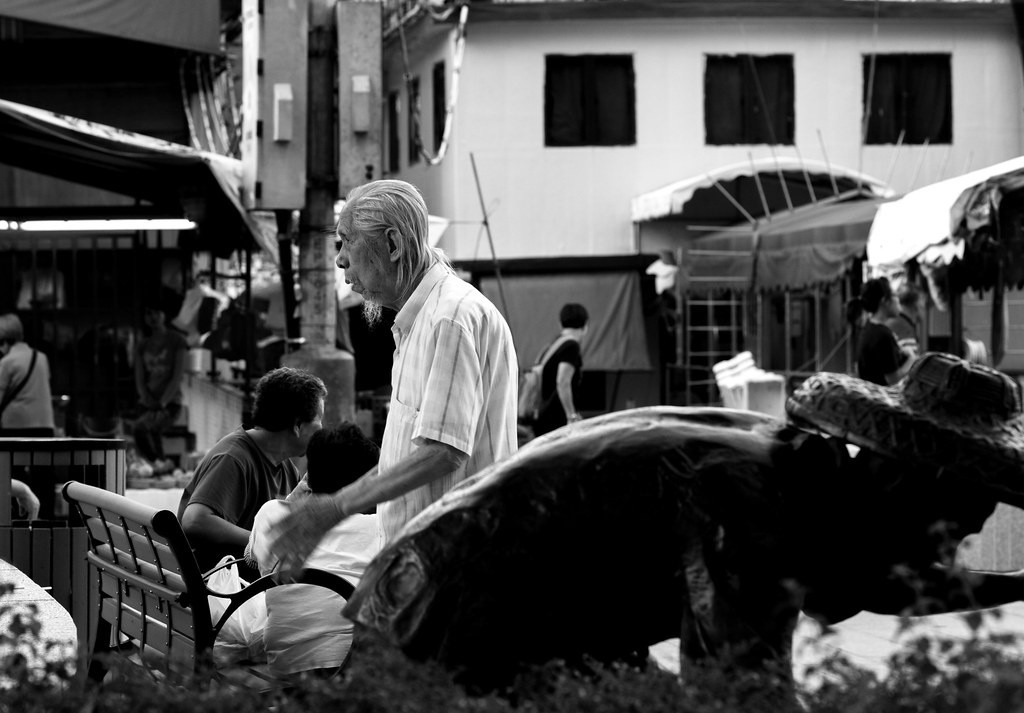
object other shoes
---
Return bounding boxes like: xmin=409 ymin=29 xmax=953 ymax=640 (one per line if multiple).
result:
xmin=154 ymin=459 xmax=174 ymax=472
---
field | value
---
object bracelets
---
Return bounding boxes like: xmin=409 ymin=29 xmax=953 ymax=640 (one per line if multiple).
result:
xmin=567 ymin=414 xmax=578 ymax=418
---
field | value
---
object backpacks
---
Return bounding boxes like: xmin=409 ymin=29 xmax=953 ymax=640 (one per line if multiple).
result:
xmin=518 ymin=334 xmax=578 ymax=425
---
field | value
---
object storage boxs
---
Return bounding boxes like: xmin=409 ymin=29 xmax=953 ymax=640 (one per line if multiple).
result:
xmin=710 ymin=350 xmax=789 ymax=422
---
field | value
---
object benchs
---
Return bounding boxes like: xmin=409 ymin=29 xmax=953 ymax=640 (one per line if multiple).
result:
xmin=61 ymin=480 xmax=355 ymax=685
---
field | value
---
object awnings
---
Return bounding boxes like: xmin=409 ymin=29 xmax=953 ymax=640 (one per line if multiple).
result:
xmin=680 ymin=156 xmax=1024 ymax=294
xmin=0 ymin=98 xmax=264 ymax=261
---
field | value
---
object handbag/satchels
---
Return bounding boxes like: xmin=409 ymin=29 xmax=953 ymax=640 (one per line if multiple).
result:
xmin=205 ymin=555 xmax=273 ymax=667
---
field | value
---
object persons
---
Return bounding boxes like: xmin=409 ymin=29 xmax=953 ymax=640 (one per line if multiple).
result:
xmin=177 ymin=368 xmax=328 ymax=573
xmin=0 ymin=314 xmax=55 ymax=438
xmin=334 ymin=351 xmax=1024 ymax=713
xmin=133 ymin=304 xmax=187 ymax=475
xmin=243 ymin=422 xmax=377 ymax=687
xmin=265 ymin=179 xmax=519 ymax=577
xmin=532 ymin=303 xmax=590 ymax=436
xmin=847 ymin=277 xmax=923 ymax=387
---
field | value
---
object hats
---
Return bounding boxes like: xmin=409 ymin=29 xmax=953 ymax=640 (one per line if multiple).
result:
xmin=789 ymin=350 xmax=1024 ymax=510
